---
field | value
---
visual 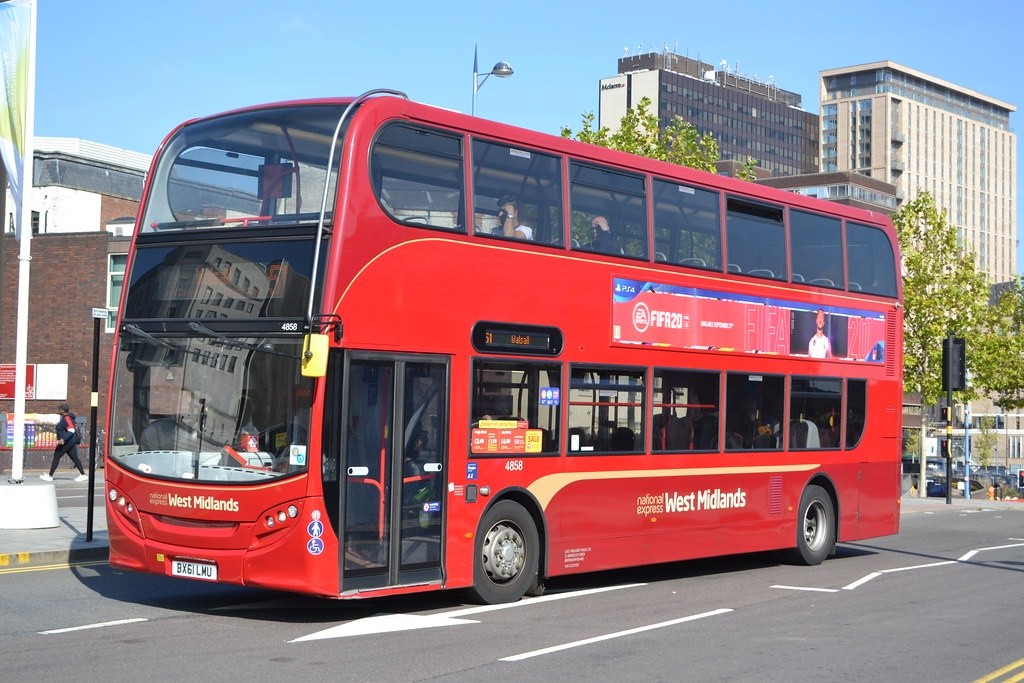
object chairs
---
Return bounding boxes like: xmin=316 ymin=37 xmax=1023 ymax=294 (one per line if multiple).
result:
xmin=403 ymin=215 xmax=863 ymax=294
xmin=534 ymin=414 xmax=841 ymax=453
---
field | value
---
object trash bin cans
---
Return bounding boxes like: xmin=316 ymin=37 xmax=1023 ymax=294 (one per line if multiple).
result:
xmin=994 ymin=487 xmax=1002 ymax=501
xmin=987 ymin=487 xmax=995 ymax=500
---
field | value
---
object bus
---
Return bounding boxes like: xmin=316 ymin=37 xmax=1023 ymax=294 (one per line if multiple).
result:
xmin=104 ymin=89 xmax=907 ymax=608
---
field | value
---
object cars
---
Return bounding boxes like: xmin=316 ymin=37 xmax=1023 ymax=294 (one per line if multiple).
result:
xmin=901 ymin=458 xmax=1018 ymax=499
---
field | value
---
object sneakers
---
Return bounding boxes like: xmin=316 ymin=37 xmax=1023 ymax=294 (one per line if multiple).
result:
xmin=75 ymin=473 xmax=88 ymax=481
xmin=40 ymin=473 xmax=54 ymax=481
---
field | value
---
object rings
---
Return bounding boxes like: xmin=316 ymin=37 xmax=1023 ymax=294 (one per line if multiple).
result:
xmin=62 ymin=443 xmax=63 ymax=445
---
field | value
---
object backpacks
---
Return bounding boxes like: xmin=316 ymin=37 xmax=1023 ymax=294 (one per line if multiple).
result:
xmin=74 ymin=423 xmax=82 ymax=444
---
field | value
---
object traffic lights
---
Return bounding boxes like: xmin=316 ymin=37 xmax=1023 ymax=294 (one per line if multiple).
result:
xmin=1018 ymin=470 xmax=1024 ymax=488
xmin=941 ymin=408 xmax=947 ymax=421
xmin=941 ymin=441 xmax=947 ymax=457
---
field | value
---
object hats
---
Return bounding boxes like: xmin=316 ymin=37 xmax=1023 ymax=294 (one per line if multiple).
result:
xmin=58 ymin=404 xmax=69 ymax=413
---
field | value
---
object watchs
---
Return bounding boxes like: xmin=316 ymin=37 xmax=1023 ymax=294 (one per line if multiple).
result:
xmin=504 ymin=215 xmax=515 ymax=219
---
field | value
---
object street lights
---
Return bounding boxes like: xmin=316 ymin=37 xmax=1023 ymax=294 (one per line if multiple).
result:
xmin=471 ymin=42 xmax=514 ymax=119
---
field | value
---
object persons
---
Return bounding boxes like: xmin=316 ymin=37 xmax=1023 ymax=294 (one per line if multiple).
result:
xmin=39 ymin=403 xmax=88 ymax=482
xmin=490 ymin=194 xmax=534 ymax=241
xmin=580 ymin=215 xmax=621 ymax=255
xmin=807 ymin=308 xmax=833 ymax=359
